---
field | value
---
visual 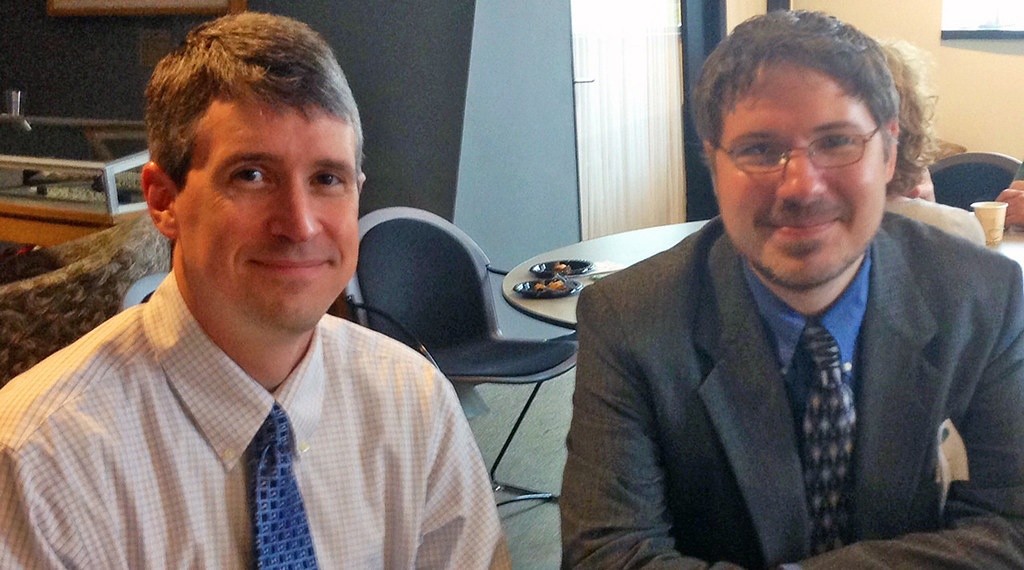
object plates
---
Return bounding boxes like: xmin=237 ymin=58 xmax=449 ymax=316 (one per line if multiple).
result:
xmin=513 ymin=278 xmax=584 ymax=298
xmin=529 ymin=259 xmax=593 ymax=279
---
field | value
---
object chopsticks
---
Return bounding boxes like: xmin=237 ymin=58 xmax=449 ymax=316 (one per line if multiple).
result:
xmin=557 ymin=273 xmax=579 ymax=291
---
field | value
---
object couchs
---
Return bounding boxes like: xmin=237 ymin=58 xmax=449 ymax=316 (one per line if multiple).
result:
xmin=0 ymin=211 xmax=171 ymax=389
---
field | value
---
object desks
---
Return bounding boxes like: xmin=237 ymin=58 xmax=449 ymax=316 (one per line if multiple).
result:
xmin=501 ymin=219 xmax=710 ymax=332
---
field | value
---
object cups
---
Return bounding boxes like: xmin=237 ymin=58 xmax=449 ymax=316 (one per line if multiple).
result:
xmin=970 ymin=201 xmax=1009 ymax=243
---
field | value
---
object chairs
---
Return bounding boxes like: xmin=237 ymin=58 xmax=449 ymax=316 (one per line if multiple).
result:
xmin=928 ymin=151 xmax=1022 ymax=211
xmin=342 ymin=205 xmax=578 ymax=507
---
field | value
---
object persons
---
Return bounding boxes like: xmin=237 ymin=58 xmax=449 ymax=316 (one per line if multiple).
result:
xmin=878 ymin=42 xmax=988 ymax=244
xmin=996 ymin=162 xmax=1024 ymax=231
xmin=559 ymin=9 xmax=1024 ymax=570
xmin=0 ymin=12 xmax=510 ymax=570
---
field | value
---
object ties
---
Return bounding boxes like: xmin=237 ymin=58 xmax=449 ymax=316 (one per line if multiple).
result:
xmin=242 ymin=402 xmax=321 ymax=570
xmin=784 ymin=318 xmax=858 ymax=557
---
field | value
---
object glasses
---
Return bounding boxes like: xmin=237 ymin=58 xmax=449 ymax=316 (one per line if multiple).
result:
xmin=708 ymin=123 xmax=882 ymax=175
